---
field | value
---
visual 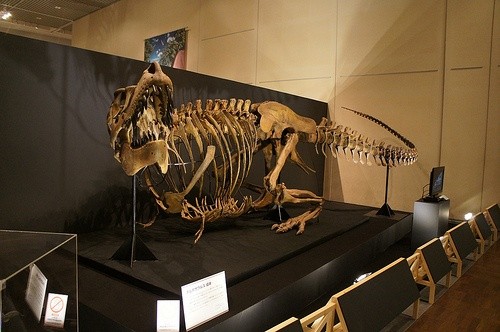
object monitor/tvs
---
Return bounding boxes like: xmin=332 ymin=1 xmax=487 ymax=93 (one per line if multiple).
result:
xmin=425 ymin=166 xmax=444 ymax=202
